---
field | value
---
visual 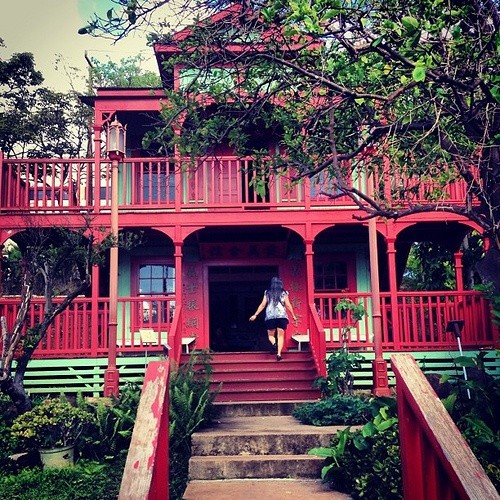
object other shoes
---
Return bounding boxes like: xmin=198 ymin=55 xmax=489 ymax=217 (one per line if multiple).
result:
xmin=272 ymin=343 xmax=276 ymax=355
xmin=277 ymin=355 xmax=283 ymax=359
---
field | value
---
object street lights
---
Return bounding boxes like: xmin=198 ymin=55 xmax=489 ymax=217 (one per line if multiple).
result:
xmin=105 ymin=112 xmax=126 ymax=400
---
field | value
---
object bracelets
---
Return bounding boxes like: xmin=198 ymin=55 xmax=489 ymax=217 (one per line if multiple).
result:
xmin=292 ymin=316 xmax=296 ymax=319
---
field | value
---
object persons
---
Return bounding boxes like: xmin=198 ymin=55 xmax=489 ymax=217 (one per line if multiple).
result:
xmin=248 ymin=277 xmax=298 ymax=361
